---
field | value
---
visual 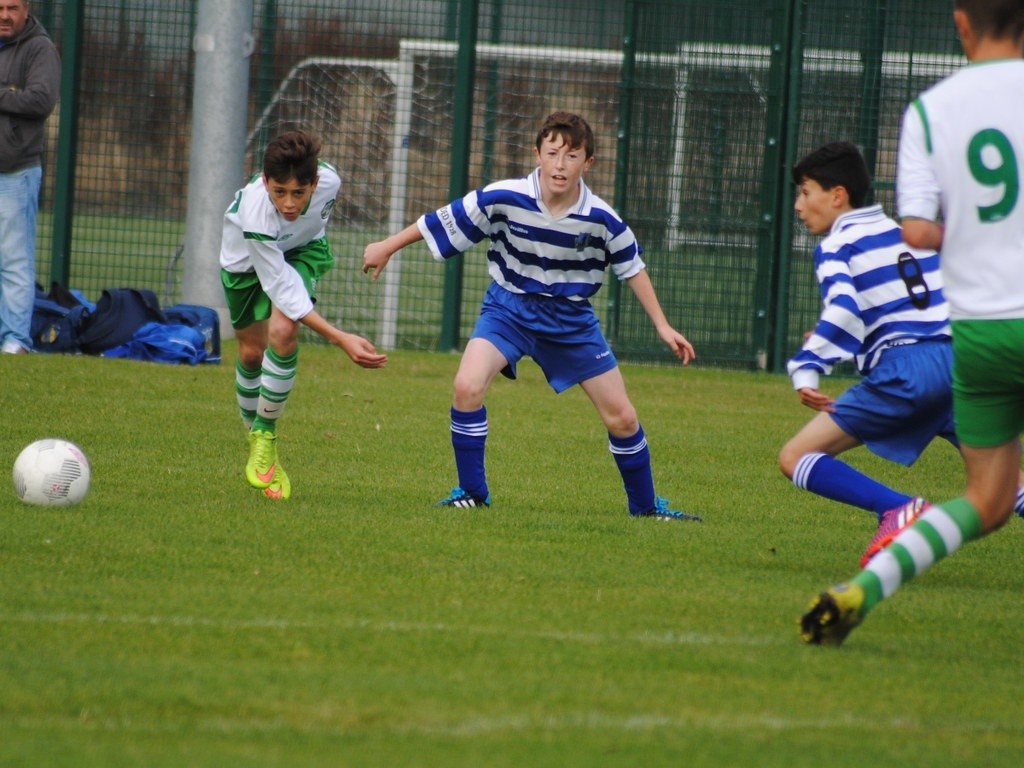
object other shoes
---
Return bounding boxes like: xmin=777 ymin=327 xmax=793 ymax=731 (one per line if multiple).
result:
xmin=2 ymin=341 xmax=26 ymax=355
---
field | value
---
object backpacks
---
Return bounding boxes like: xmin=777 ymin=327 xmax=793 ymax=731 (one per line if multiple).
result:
xmin=57 ymin=286 xmax=172 ymax=356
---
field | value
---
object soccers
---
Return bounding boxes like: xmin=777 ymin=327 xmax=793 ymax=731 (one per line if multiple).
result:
xmin=11 ymin=436 xmax=92 ymax=508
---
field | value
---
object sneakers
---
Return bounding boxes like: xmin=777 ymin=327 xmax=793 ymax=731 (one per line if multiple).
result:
xmin=433 ymin=489 xmax=491 ymax=510
xmin=860 ymin=496 xmax=931 ymax=569
xmin=245 ymin=426 xmax=277 ymax=489
xmin=260 ymin=458 xmax=291 ymax=501
xmin=628 ymin=507 xmax=703 ymax=523
xmin=795 ymin=580 xmax=864 ymax=649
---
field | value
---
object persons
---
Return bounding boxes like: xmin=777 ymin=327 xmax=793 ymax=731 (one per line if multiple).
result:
xmin=362 ymin=112 xmax=704 ymax=523
xmin=218 ymin=130 xmax=388 ymax=501
xmin=1 ymin=0 xmax=59 ymax=354
xmin=799 ymin=0 xmax=1023 ymax=640
xmin=777 ymin=141 xmax=1024 ymax=571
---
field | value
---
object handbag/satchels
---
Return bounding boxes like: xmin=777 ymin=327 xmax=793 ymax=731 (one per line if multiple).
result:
xmin=160 ymin=301 xmax=222 ymax=365
xmin=27 ymin=279 xmax=96 ymax=352
